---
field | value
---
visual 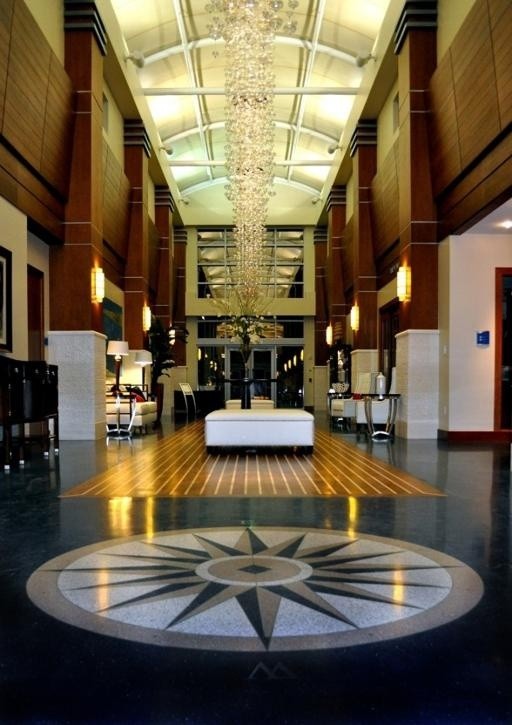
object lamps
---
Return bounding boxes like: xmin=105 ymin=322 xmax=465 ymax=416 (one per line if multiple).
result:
xmin=121 ymin=49 xmax=145 ymax=69
xmin=105 ymin=340 xmax=133 ymax=395
xmin=94 ymin=267 xmax=105 ymax=302
xmin=177 ymin=197 xmax=190 ymax=205
xmin=158 ymin=141 xmax=174 ymax=158
xmin=325 ymin=326 xmax=332 ymax=346
xmin=396 ymin=266 xmax=407 ymax=302
xmin=350 ymin=305 xmax=360 ymax=332
xmin=133 ymin=350 xmax=153 ymax=393
xmin=355 ymin=50 xmax=377 ymax=67
xmin=312 ymin=196 xmax=324 ymax=204
xmin=202 ymin=0 xmax=299 ymax=318
xmin=328 ymin=142 xmax=344 ymax=154
xmin=142 ymin=306 xmax=151 ymax=332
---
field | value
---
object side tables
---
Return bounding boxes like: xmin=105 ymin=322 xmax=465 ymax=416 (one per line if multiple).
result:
xmin=104 ymin=394 xmax=137 ymax=448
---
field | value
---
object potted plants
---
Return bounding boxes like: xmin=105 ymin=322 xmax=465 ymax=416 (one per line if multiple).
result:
xmin=144 ymin=317 xmax=190 ymax=423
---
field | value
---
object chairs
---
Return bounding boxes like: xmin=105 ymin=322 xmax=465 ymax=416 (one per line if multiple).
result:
xmin=327 ymin=393 xmax=398 ymax=443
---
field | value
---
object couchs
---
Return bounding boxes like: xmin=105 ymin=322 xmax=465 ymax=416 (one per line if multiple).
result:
xmin=105 ymin=401 xmax=158 ymax=435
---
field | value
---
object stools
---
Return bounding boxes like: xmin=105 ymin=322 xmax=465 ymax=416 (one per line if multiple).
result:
xmin=205 ymin=409 xmax=314 ymax=455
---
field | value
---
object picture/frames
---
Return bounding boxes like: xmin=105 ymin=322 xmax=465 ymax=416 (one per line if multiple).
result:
xmin=0 ymin=245 xmax=13 ymax=354
